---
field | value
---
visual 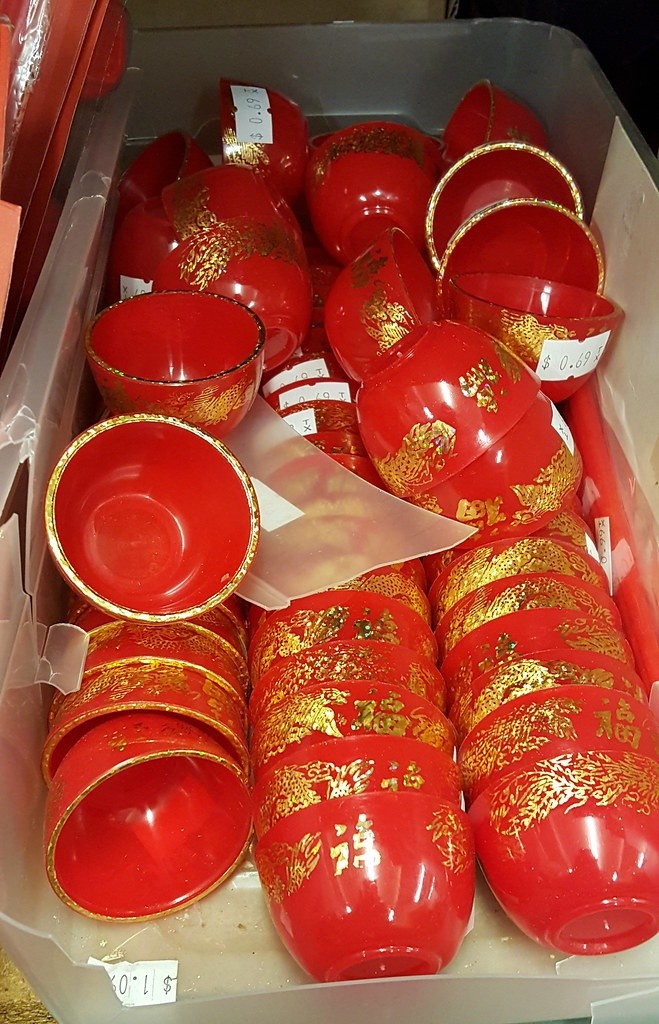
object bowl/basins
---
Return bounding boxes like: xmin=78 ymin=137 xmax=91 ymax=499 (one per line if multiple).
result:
xmin=38 ymin=78 xmax=659 ymax=983
